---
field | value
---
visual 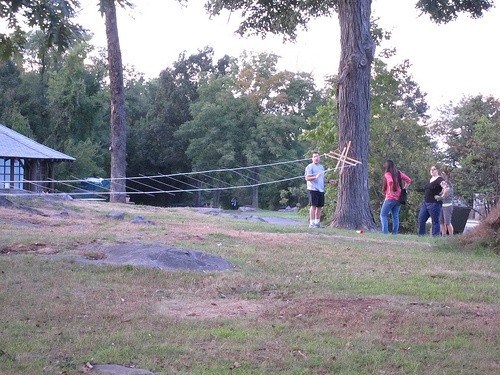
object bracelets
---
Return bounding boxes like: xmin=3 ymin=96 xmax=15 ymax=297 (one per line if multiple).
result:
xmin=328 ymin=180 xmax=329 ymax=183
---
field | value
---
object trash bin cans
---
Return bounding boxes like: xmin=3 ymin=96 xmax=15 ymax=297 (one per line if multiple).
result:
xmin=451 ymin=205 xmax=472 ymax=234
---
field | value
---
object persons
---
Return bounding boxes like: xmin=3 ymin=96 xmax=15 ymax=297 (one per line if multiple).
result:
xmin=231 ymin=197 xmax=237 ymax=209
xmin=305 ymin=152 xmax=337 ymax=228
xmin=417 ymin=165 xmax=454 ymax=238
xmin=379 ymin=159 xmax=412 ymax=235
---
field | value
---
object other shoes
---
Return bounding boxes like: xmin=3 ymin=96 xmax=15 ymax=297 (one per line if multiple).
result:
xmin=309 ymin=222 xmax=320 ymax=228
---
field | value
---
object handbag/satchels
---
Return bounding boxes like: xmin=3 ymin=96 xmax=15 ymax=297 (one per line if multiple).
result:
xmin=400 ymin=189 xmax=406 ymax=204
